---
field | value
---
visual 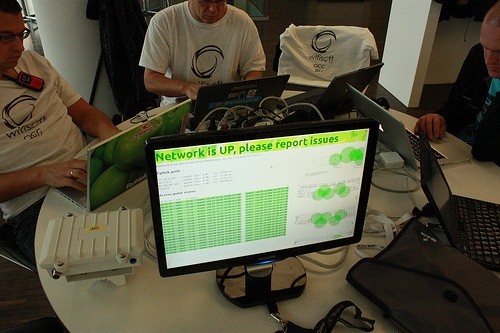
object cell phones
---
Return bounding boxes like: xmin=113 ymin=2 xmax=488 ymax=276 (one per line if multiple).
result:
xmin=2 ymin=71 xmax=44 ymax=91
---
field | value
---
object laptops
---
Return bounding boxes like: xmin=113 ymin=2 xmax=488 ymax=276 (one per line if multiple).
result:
xmin=346 ymin=82 xmax=473 ymax=170
xmin=175 ymin=74 xmax=290 ymax=131
xmin=52 ymin=98 xmax=191 ymax=213
xmin=277 ymin=63 xmax=385 ymax=122
xmin=418 ymin=122 xmax=500 ymax=272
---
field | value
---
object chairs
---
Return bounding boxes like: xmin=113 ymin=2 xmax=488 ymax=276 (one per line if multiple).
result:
xmin=272 ymin=22 xmax=379 ymax=102
xmin=0 ymin=219 xmax=69 ymax=333
xmin=85 ymin=0 xmax=163 ymax=129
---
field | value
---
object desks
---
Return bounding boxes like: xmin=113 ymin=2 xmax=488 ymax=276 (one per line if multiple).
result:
xmin=35 ymin=91 xmax=500 ymax=333
xmin=143 ymin=0 xmax=270 ymax=26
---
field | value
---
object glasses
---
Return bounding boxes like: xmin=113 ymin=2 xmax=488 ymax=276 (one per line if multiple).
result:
xmin=0 ymin=27 xmax=31 ymax=44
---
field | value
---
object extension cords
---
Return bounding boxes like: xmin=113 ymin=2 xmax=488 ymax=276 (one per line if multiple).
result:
xmin=199 ymin=120 xmax=274 ymax=132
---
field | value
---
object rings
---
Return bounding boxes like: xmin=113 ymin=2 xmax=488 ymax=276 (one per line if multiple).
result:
xmin=70 ymin=169 xmax=75 ymax=177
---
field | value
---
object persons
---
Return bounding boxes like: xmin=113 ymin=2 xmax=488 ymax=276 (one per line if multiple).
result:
xmin=138 ymin=0 xmax=266 ymax=101
xmin=414 ymin=0 xmax=500 ymax=162
xmin=0 ymin=0 xmax=120 ymax=266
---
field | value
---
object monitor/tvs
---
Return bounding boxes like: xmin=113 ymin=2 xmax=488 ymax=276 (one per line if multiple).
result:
xmin=144 ymin=116 xmax=379 ymax=308
